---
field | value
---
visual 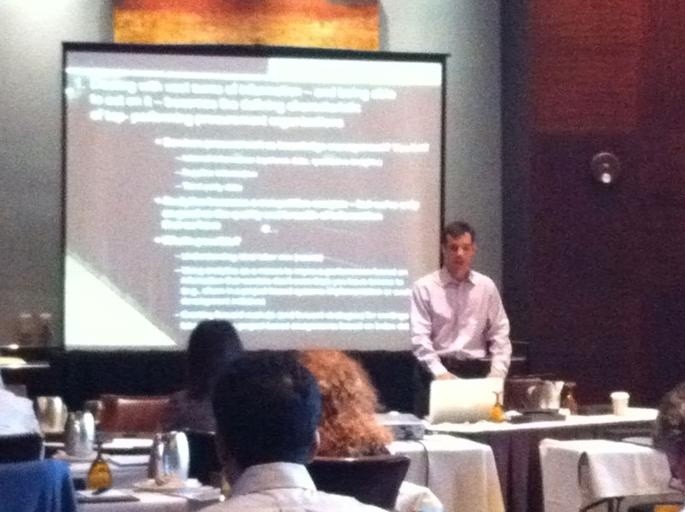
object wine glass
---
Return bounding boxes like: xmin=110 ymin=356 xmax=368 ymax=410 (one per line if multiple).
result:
xmin=490 ymin=389 xmax=505 ymax=423
xmin=83 ymin=439 xmax=114 ymax=489
xmin=563 ymin=380 xmax=580 ymax=416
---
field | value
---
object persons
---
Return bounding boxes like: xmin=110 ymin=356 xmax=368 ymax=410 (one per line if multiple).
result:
xmin=290 ymin=349 xmax=447 ymax=512
xmin=161 ymin=321 xmax=246 ymax=432
xmin=197 ymin=350 xmax=389 ymax=512
xmin=409 ymin=220 xmax=513 ymax=419
xmin=653 ymin=382 xmax=685 ymax=512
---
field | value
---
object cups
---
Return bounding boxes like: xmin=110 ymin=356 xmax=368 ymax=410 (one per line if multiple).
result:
xmin=149 ymin=430 xmax=190 ymax=481
xmin=611 ymin=390 xmax=630 ymax=416
xmin=64 ymin=409 xmax=96 ymax=456
xmin=37 ymin=396 xmax=68 ymax=437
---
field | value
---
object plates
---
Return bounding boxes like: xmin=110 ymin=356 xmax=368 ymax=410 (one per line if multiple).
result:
xmin=130 ymin=477 xmax=202 ymax=492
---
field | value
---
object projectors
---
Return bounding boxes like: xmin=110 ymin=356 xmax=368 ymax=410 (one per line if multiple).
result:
xmin=374 ymin=412 xmax=424 ymax=440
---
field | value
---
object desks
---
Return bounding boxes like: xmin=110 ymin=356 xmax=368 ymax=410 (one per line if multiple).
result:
xmin=0 ymin=395 xmax=685 ymax=512
xmin=0 ymin=360 xmax=52 ymax=384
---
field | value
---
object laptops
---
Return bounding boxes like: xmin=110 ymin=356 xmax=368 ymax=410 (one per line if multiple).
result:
xmin=423 ymin=378 xmax=504 ymax=424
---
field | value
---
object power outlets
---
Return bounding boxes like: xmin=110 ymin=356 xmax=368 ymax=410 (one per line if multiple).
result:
xmin=38 ymin=314 xmax=53 ymax=348
xmin=18 ymin=314 xmax=34 ymax=348
